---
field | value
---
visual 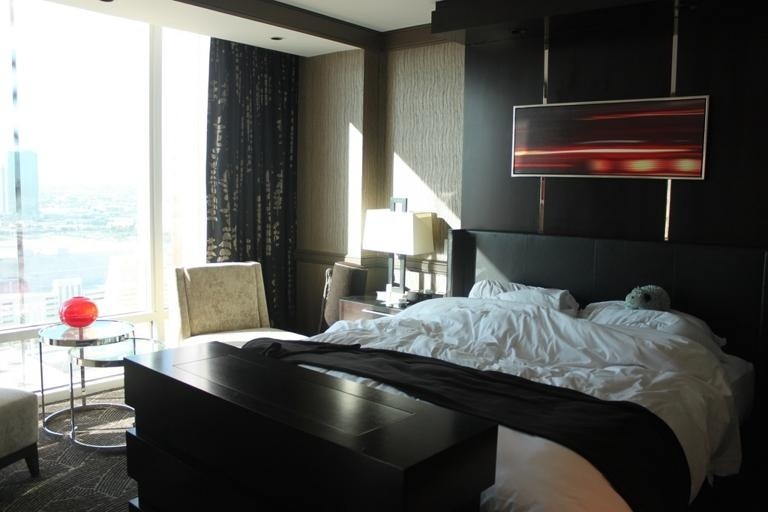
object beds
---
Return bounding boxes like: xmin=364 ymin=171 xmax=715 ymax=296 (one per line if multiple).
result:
xmin=298 ymin=228 xmax=757 ymax=512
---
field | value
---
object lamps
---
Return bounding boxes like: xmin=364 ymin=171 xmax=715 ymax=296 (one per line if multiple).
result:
xmin=362 ymin=195 xmax=436 ymax=310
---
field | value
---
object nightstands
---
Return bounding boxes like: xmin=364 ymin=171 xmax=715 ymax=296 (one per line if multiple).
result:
xmin=337 ymin=290 xmax=414 ymax=322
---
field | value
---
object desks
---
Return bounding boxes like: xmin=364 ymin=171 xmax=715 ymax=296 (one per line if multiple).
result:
xmin=124 ymin=339 xmax=498 ymax=512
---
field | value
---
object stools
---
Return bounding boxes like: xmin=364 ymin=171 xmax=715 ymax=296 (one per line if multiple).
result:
xmin=0 ymin=388 xmax=40 ymax=479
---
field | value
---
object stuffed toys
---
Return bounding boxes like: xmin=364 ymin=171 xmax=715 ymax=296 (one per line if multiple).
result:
xmin=625 ymin=283 xmax=674 ymax=311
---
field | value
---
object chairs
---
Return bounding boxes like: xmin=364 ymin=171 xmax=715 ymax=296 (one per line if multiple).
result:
xmin=175 ymin=260 xmax=271 ymax=339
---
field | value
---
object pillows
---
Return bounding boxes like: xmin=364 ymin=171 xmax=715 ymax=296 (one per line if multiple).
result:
xmin=468 ymin=278 xmax=579 ymax=316
xmin=582 ymin=301 xmax=729 ymax=363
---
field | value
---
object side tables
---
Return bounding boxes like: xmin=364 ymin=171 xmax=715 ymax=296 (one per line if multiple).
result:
xmin=37 ymin=318 xmax=165 ymax=456
